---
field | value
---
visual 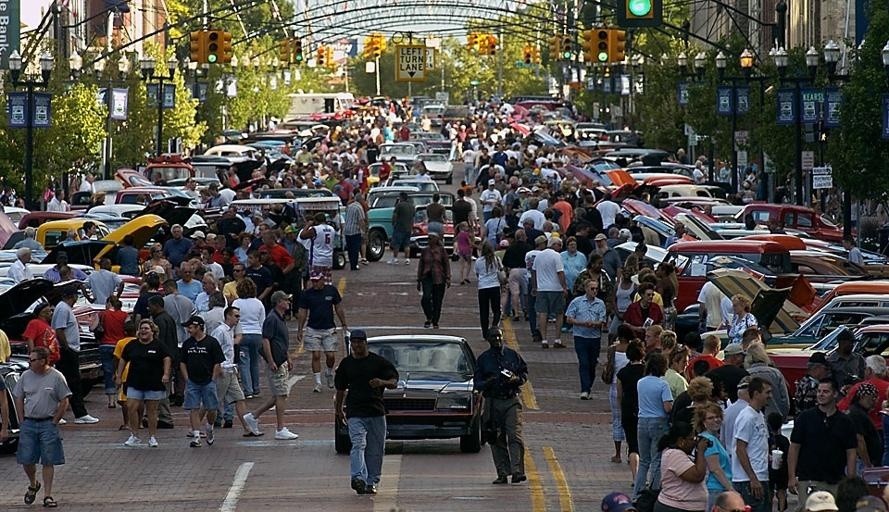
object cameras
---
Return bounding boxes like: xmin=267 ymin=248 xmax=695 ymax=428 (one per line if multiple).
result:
xmin=695 ymin=436 xmax=713 ymax=450
xmin=497 ymin=367 xmax=511 ymax=385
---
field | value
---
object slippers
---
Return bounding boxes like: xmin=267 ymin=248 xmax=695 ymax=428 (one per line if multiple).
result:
xmin=243 ymin=432 xmax=264 ymax=436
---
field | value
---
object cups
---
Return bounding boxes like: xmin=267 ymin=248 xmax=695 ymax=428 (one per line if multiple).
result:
xmin=771 ymin=449 xmax=784 ymax=469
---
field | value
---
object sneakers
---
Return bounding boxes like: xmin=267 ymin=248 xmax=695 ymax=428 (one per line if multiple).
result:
xmin=148 ymin=437 xmax=159 ymax=447
xmin=404 ymin=258 xmax=410 ymax=263
xmin=313 ymin=383 xmax=323 ymax=393
xmin=357 ymin=257 xmax=369 ymax=266
xmin=424 ymin=321 xmax=431 ymax=327
xmin=242 ymin=413 xmax=259 ymax=435
xmin=580 ymin=392 xmax=593 ymax=400
xmin=74 ymin=415 xmax=100 ymax=424
xmin=275 ymin=428 xmax=299 ymax=440
xmin=386 ymin=258 xmax=399 ymax=265
xmin=187 ymin=419 xmax=233 ymax=447
xmin=325 ymin=369 xmax=334 ymax=388
xmin=125 ymin=433 xmax=142 ymax=444
xmin=434 ymin=323 xmax=439 ymax=327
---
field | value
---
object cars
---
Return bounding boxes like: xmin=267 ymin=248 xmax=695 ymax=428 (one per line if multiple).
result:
xmin=0 ymin=90 xmax=349 ymax=457
xmin=360 ymin=98 xmax=483 ymax=264
xmin=330 ymin=328 xmax=490 ymax=457
xmin=504 ymin=91 xmax=889 ymax=419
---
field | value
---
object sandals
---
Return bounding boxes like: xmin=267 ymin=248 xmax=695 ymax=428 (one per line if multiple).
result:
xmin=24 ymin=480 xmax=41 ymax=504
xmin=42 ymin=496 xmax=57 ymax=507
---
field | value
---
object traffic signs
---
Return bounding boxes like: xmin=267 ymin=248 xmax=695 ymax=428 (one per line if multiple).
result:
xmin=423 ymin=46 xmax=436 ymax=71
xmin=396 ymin=43 xmax=428 ymax=82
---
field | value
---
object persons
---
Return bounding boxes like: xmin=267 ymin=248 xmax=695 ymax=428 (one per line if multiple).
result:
xmin=416 ymin=232 xmax=452 ymax=329
xmin=426 ymin=194 xmax=448 ymax=247
xmin=440 ymin=100 xmax=884 ymax=511
xmin=12 ymin=346 xmax=73 ymax=507
xmin=334 ymin=329 xmax=399 ymax=495
xmin=472 ymin=327 xmax=528 ymax=485
xmin=2 ymin=98 xmax=431 ymax=448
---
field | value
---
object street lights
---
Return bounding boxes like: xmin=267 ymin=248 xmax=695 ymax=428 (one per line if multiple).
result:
xmin=716 ymin=50 xmax=744 ymax=196
xmin=882 ymin=41 xmax=889 ymax=78
xmin=69 ymin=50 xmax=104 ymax=88
xmin=565 ymin=50 xmax=709 ymax=161
xmin=822 ymin=39 xmax=868 ymax=239
xmin=8 ymin=49 xmax=54 ymax=211
xmin=772 ymin=46 xmax=819 ymax=207
xmin=740 ymin=46 xmax=778 ymax=196
xmin=102 ymin=55 xmax=285 ymax=179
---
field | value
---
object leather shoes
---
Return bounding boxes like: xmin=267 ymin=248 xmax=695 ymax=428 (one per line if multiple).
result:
xmin=554 ymin=342 xmax=565 ymax=348
xmin=348 ymin=477 xmax=376 ymax=493
xmin=493 ymin=475 xmax=508 ymax=483
xmin=541 ymin=344 xmax=548 ymax=348
xmin=511 ymin=473 xmax=526 ymax=483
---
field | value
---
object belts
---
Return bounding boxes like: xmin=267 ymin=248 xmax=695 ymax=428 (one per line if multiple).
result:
xmin=24 ymin=417 xmax=52 ymax=422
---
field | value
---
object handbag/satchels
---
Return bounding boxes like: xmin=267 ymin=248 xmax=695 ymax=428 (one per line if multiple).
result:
xmin=496 ymin=269 xmax=506 ymax=283
xmin=602 ymin=362 xmax=614 ymax=384
xmin=633 ymin=488 xmax=662 ymax=510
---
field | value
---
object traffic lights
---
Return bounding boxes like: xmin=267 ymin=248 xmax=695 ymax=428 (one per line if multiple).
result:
xmin=616 ymin=0 xmax=663 ymax=27
xmin=206 ymin=31 xmax=219 ymax=63
xmin=218 ymin=30 xmax=232 ymax=64
xmin=279 ymin=30 xmax=628 ymax=67
xmin=189 ymin=30 xmax=205 ymax=63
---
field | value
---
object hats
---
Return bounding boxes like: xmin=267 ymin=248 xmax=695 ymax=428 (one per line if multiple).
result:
xmin=534 ymin=236 xmax=547 ymax=245
xmin=349 ymin=330 xmax=367 ymax=339
xmin=182 ymin=316 xmax=205 ymax=327
xmin=34 ymin=301 xmax=48 ymax=315
xmin=723 ymin=343 xmax=745 ymax=356
xmin=810 ymin=352 xmax=831 ymax=366
xmin=804 ymin=492 xmax=837 ymax=512
xmin=309 ymin=271 xmax=325 ymax=281
xmin=855 ymin=494 xmax=887 ymax=512
xmin=60 ymin=286 xmax=81 ymax=295
xmin=270 ymin=291 xmax=292 ymax=305
xmin=601 ymin=492 xmax=637 ymax=512
xmin=594 ymin=234 xmax=607 ymax=241
xmin=836 ymin=329 xmax=859 ymax=341
